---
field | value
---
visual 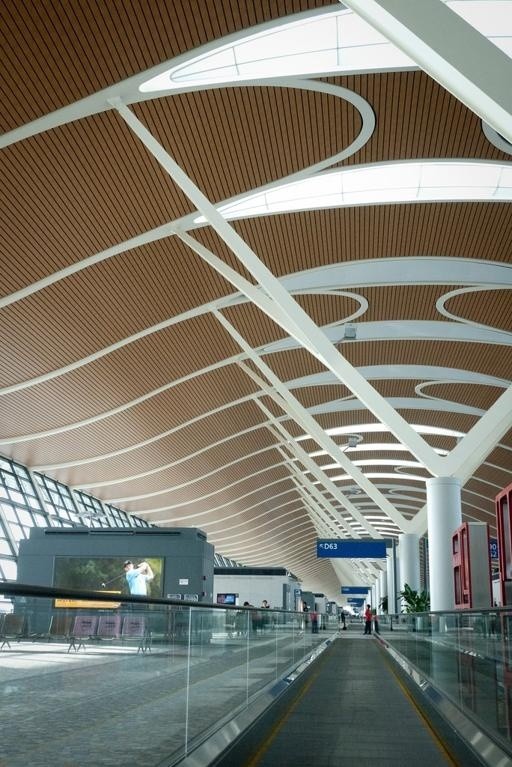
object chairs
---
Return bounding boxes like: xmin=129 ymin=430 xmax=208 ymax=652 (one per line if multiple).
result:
xmin=0 ymin=612 xmax=151 ymax=657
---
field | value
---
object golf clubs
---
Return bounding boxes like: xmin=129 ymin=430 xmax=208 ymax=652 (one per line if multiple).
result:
xmin=102 ymin=567 xmax=138 ymax=588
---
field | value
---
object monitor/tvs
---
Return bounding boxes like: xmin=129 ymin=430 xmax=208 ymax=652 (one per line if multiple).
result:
xmin=51 ymin=556 xmax=164 ymax=605
xmin=216 ymin=593 xmax=237 ymax=605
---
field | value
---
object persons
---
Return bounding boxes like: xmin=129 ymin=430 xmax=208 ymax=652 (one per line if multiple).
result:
xmin=261 ymin=600 xmax=269 ymax=608
xmin=217 ymin=595 xmax=226 ymax=603
xmin=363 ymin=604 xmax=371 ymax=634
xmin=123 ymin=560 xmax=153 ymax=596
xmin=312 ymin=613 xmax=318 ymax=633
xmin=244 ymin=602 xmax=251 ymax=607
xmin=341 ymin=611 xmax=345 ymax=622
xmin=373 ymin=615 xmax=378 ymax=633
xmin=304 ymin=602 xmax=307 ymax=611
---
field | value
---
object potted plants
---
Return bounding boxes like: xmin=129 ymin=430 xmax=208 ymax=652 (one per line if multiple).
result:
xmin=395 ymin=583 xmax=430 ymax=630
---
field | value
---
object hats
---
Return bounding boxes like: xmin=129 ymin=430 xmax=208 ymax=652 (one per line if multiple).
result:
xmin=124 ymin=560 xmax=132 ymax=567
